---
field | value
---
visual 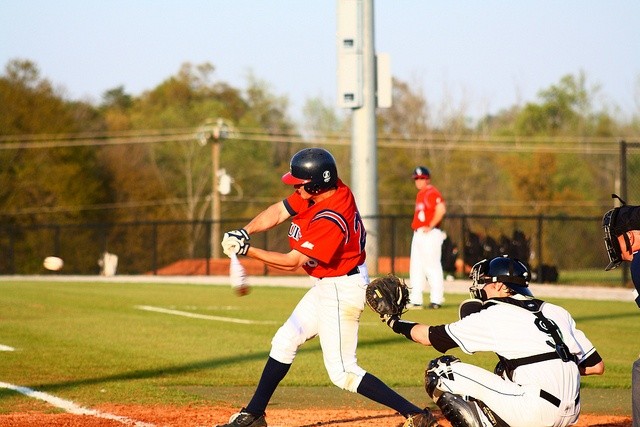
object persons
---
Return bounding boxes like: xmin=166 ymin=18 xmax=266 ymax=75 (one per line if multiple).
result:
xmin=363 ymin=253 xmax=606 ymax=426
xmin=210 ymin=145 xmax=443 ymax=425
xmin=404 ymin=165 xmax=449 ymax=309
xmin=602 ymin=205 xmax=639 ymax=426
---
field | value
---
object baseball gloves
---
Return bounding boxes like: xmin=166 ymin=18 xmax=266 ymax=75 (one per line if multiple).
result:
xmin=363 ymin=272 xmax=413 ymax=322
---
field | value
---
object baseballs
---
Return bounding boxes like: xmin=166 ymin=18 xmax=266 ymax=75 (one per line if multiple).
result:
xmin=43 ymin=256 xmax=64 ymax=271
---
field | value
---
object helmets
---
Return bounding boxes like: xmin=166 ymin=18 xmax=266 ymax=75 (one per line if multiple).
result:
xmin=281 ymin=147 xmax=338 ymax=194
xmin=469 ymin=255 xmax=533 ymax=300
xmin=603 ymin=205 xmax=640 ymax=271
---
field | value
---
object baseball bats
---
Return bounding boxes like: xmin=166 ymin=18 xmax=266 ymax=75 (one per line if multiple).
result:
xmin=229 ymin=246 xmax=250 ymax=297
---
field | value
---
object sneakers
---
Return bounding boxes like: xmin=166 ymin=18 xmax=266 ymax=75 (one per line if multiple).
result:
xmin=403 ymin=407 xmax=441 ymax=426
xmin=216 ymin=408 xmax=267 ymax=427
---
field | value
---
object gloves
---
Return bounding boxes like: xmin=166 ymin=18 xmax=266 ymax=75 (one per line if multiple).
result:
xmin=222 ymin=228 xmax=250 ymax=256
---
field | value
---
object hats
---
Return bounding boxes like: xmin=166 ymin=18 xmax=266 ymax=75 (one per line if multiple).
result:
xmin=412 ymin=166 xmax=430 ymax=179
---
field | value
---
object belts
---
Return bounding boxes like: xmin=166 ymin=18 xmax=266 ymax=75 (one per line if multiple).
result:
xmin=540 ymin=389 xmax=580 ymax=409
